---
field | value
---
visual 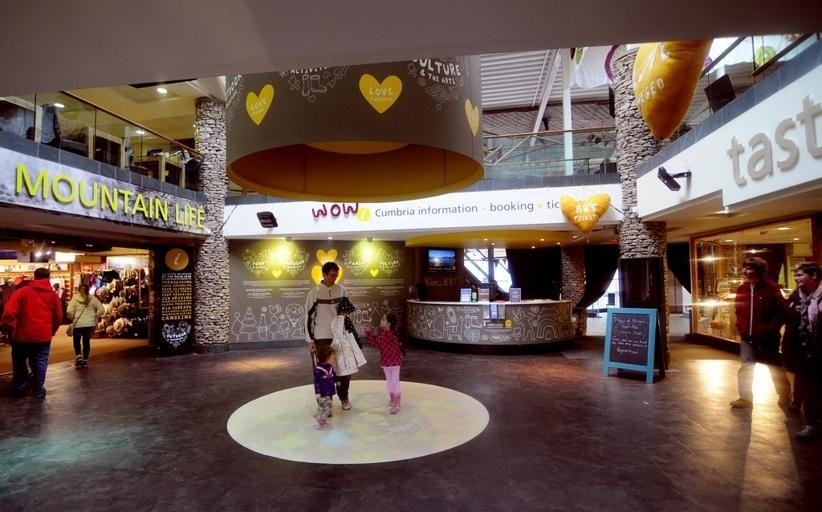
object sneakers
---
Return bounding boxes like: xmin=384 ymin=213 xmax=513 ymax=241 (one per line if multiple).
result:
xmin=341 ymin=398 xmax=351 ymax=410
xmin=75 ymin=357 xmax=88 ymax=367
xmin=731 ymin=398 xmax=752 ymax=409
xmin=778 ymin=395 xmax=800 ymax=411
xmin=389 ymin=401 xmax=400 ymax=414
xmin=34 ymin=388 xmax=45 ymax=397
xmin=796 ymin=425 xmax=814 ymax=436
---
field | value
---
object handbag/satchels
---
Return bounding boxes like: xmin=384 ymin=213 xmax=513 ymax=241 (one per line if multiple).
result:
xmin=67 ymin=324 xmax=73 ymax=336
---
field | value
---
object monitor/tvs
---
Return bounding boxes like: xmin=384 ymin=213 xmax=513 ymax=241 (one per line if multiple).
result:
xmin=416 ymin=282 xmax=428 ymax=301
xmin=425 ymin=248 xmax=458 ymax=274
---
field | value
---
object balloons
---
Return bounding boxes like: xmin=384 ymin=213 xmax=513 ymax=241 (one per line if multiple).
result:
xmin=560 ymin=193 xmax=610 ymax=235
xmin=631 ymin=40 xmax=714 ymax=141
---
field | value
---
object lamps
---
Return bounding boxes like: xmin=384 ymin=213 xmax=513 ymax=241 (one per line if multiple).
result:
xmin=255 ymin=211 xmax=278 ymax=229
xmin=653 ymin=164 xmax=693 ymax=194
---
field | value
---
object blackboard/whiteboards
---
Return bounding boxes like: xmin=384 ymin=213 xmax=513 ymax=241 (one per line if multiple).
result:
xmin=603 ymin=308 xmax=657 ymax=372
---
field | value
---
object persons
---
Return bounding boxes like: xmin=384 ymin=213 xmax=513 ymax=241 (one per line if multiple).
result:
xmin=0 ymin=267 xmax=65 ymax=399
xmin=363 ymin=312 xmax=404 ymax=415
xmin=727 ymin=255 xmax=792 ymax=410
xmin=66 ymin=283 xmax=106 ymax=369
xmin=52 ymin=283 xmax=64 ymax=299
xmin=779 ymin=262 xmax=821 ymax=441
xmin=310 ymin=345 xmax=342 ymax=430
xmin=304 ymin=261 xmax=353 ymax=418
xmin=180 ymin=148 xmax=201 ymax=164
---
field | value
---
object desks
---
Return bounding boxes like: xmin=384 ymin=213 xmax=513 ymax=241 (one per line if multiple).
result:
xmin=62 ymin=125 xmax=125 ymax=171
xmin=130 ymin=154 xmax=185 ymax=189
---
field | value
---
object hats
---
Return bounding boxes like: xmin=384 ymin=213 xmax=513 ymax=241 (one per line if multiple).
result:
xmin=90 ymin=280 xmax=133 ymax=338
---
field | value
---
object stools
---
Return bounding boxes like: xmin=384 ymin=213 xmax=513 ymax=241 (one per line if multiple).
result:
xmin=59 ymin=137 xmax=88 ymax=155
